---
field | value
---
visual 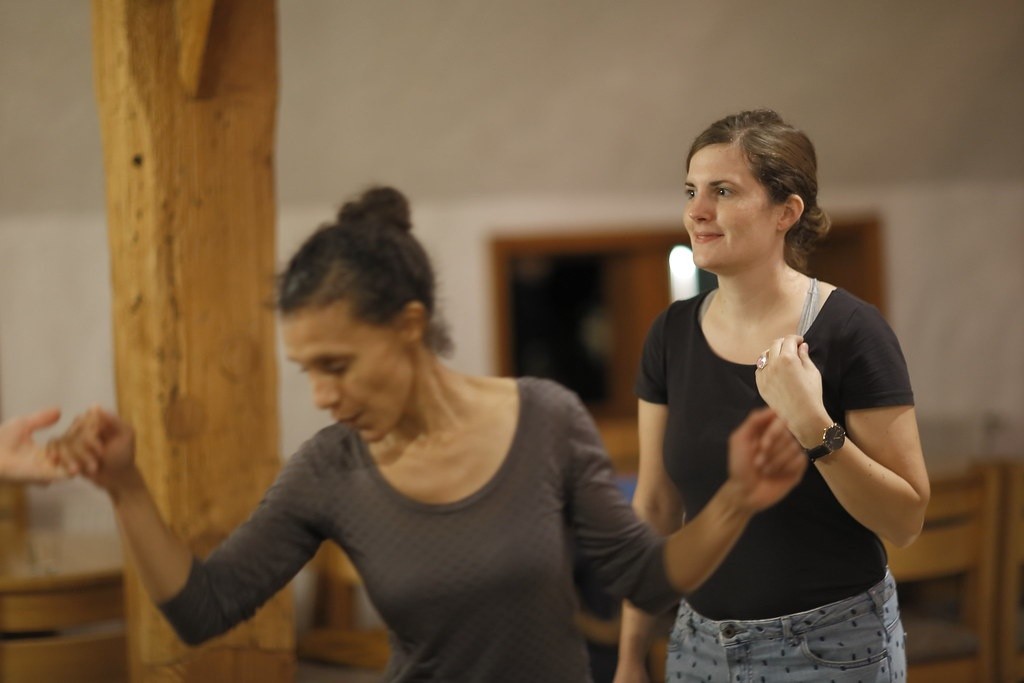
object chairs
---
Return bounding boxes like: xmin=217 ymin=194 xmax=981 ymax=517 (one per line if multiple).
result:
xmin=881 ymin=457 xmax=1003 ymax=683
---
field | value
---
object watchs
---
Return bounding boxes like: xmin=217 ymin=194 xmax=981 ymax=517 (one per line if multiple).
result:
xmin=807 ymin=423 xmax=846 ymax=461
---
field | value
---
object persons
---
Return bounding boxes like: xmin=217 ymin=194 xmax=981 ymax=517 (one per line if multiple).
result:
xmin=48 ymin=184 xmax=663 ymax=683
xmin=0 ymin=404 xmax=103 ymax=482
xmin=615 ymin=108 xmax=930 ymax=682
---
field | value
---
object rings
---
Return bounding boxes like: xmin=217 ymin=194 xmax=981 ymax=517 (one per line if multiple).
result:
xmin=756 ymin=357 xmax=768 ymax=369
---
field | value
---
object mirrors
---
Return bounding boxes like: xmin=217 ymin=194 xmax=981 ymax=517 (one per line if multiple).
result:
xmin=486 ymin=206 xmax=891 ymax=461
